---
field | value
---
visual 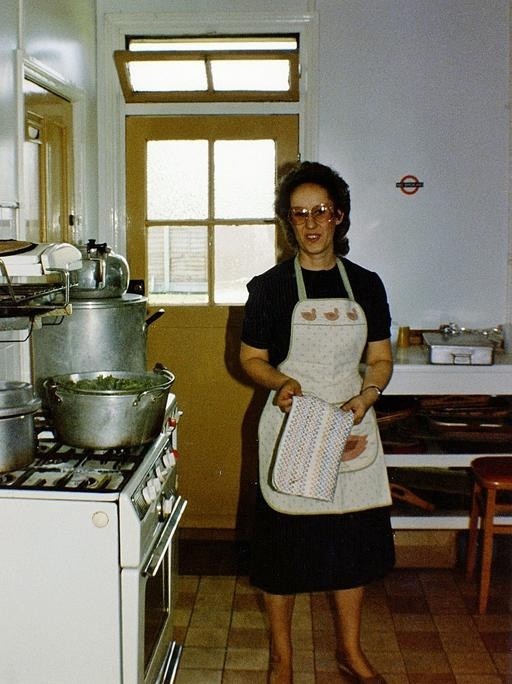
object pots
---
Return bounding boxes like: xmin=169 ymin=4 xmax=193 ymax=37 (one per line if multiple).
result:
xmin=33 ymin=292 xmax=166 ymax=401
xmin=0 ymin=379 xmax=40 ymax=477
xmin=44 ymin=367 xmax=174 ymax=450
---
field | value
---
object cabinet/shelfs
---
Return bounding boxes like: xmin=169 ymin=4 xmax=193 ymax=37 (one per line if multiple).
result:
xmin=356 ymin=338 xmax=512 ymax=530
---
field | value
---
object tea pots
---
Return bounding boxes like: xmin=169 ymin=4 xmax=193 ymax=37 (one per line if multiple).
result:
xmin=57 ymin=237 xmax=130 ymax=300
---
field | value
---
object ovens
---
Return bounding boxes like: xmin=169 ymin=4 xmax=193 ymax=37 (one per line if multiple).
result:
xmin=120 ymin=495 xmax=181 ymax=684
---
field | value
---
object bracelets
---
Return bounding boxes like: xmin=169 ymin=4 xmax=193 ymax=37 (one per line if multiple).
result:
xmin=361 ymin=383 xmax=383 ymax=400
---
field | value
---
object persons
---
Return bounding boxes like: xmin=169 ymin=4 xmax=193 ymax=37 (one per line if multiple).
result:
xmin=238 ymin=160 xmax=395 ymax=683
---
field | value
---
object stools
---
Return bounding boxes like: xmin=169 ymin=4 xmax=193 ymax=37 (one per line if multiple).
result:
xmin=463 ymin=455 xmax=512 ymax=618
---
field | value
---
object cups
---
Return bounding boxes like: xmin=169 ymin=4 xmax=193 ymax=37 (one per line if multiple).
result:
xmin=398 ymin=349 xmax=410 ymax=365
xmin=398 ymin=326 xmax=410 ymax=350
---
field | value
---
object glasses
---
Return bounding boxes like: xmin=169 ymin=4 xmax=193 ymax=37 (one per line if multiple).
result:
xmin=284 ymin=202 xmax=336 ymax=227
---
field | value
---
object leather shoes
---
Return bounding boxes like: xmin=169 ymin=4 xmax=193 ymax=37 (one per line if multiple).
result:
xmin=335 ymin=650 xmax=386 ymax=683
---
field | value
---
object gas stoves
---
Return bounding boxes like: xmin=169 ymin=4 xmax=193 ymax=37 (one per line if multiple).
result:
xmin=0 ymin=390 xmax=182 ymax=520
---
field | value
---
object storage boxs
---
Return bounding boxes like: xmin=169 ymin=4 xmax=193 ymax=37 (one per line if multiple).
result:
xmin=392 ymin=527 xmax=457 ymax=572
